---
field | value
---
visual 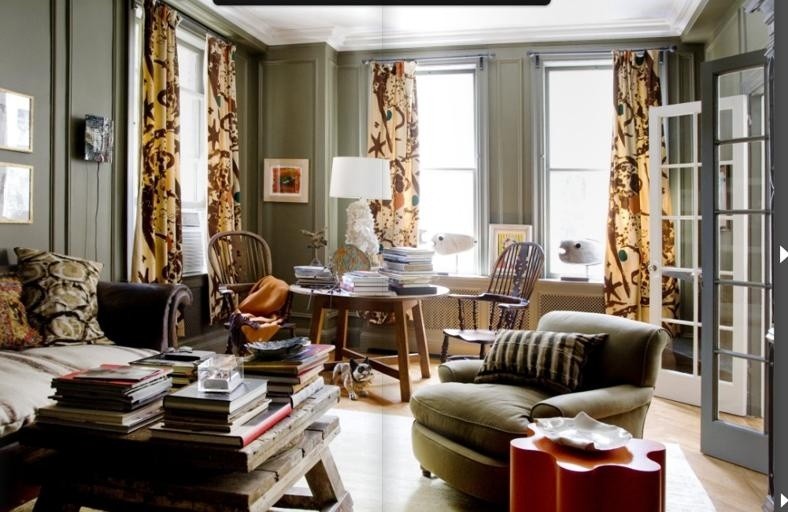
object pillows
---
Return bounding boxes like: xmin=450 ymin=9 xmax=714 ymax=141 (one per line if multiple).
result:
xmin=473 ymin=326 xmax=609 ymax=394
xmin=13 ymin=248 xmax=117 ymax=346
xmin=0 ymin=271 xmax=45 ymax=349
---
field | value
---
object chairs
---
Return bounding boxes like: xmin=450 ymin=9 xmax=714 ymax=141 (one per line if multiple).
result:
xmin=410 ymin=310 xmax=671 ymax=509
xmin=207 ymin=230 xmax=297 ymax=355
xmin=440 ymin=241 xmax=545 ymax=365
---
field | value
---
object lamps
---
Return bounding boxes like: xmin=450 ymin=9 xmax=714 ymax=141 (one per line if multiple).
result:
xmin=328 ymin=155 xmax=392 ymax=267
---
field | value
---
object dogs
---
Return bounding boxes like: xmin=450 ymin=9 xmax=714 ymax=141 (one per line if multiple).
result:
xmin=332 ymin=356 xmax=374 ymax=401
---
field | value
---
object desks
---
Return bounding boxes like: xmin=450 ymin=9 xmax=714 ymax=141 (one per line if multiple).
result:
xmin=34 ymin=411 xmax=356 ymax=512
xmin=289 ymin=282 xmax=449 ymax=402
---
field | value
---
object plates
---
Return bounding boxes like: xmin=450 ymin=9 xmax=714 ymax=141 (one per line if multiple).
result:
xmin=534 ymin=410 xmax=634 ymax=454
xmin=243 ymin=336 xmax=311 ymax=362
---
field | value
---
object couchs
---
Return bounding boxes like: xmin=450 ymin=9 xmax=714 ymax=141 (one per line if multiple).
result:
xmin=0 ymin=250 xmax=194 ymax=512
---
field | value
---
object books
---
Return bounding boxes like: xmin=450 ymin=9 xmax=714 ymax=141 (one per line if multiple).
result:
xmin=233 ymin=344 xmax=336 ymax=409
xmin=34 ymin=350 xmax=216 ymax=432
xmin=147 ymin=379 xmax=292 ymax=448
xmin=292 ymin=244 xmax=438 ymax=296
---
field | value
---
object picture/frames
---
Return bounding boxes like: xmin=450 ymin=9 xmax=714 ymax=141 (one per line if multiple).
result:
xmin=0 ymin=88 xmax=34 ymax=153
xmin=0 ymin=161 xmax=33 ymax=224
xmin=488 ymin=223 xmax=532 ymax=279
xmin=263 ymin=158 xmax=308 ymax=203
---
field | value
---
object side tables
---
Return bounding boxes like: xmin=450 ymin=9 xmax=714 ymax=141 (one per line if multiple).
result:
xmin=510 ymin=423 xmax=667 ymax=512
